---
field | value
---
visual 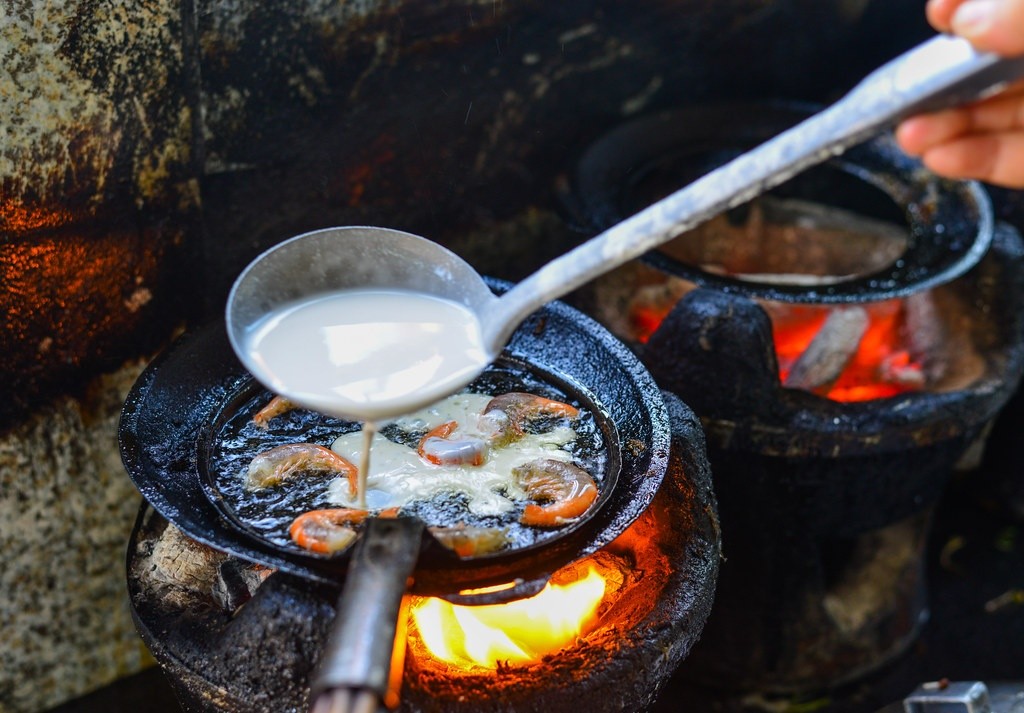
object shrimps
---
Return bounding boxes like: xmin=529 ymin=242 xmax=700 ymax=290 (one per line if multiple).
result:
xmin=238 ymin=385 xmax=596 ymax=557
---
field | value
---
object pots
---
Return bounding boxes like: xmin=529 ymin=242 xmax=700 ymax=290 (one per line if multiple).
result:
xmin=197 ymin=350 xmax=620 ymax=712
